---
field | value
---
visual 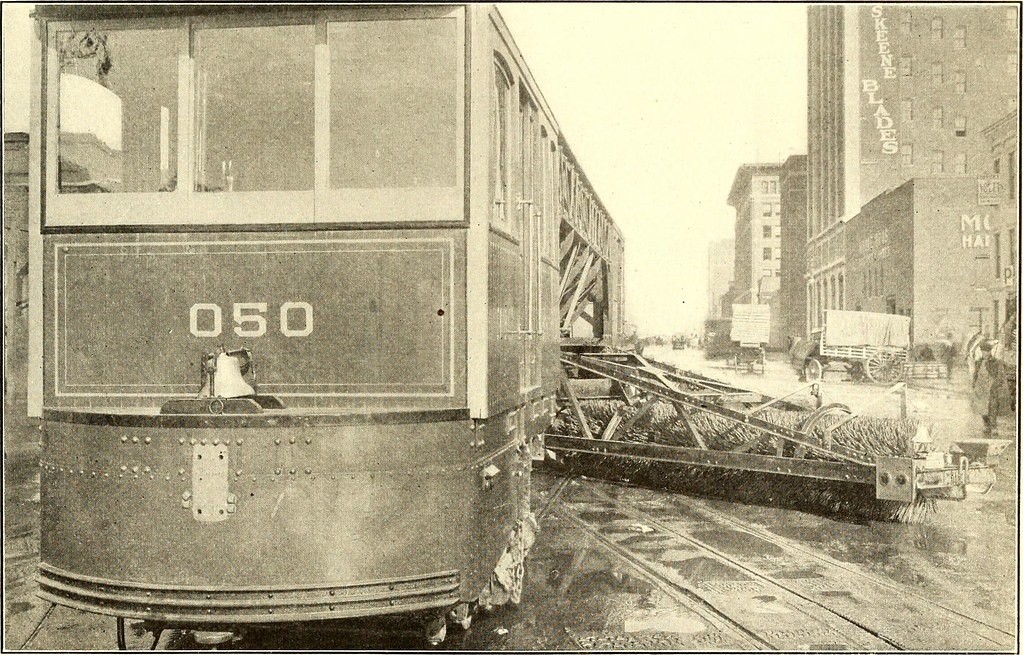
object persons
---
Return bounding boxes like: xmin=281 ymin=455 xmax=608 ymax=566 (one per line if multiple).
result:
xmin=971 ymin=343 xmax=1008 ymax=437
xmin=787 ymin=335 xmax=793 ymax=351
xmin=939 ymin=333 xmax=958 ymax=379
xmin=918 ymin=342 xmax=935 ymax=362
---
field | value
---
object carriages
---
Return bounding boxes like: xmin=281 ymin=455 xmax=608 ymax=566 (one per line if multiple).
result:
xmin=788 ymin=309 xmax=912 ymax=386
xmin=729 ymin=302 xmax=770 ymax=373
